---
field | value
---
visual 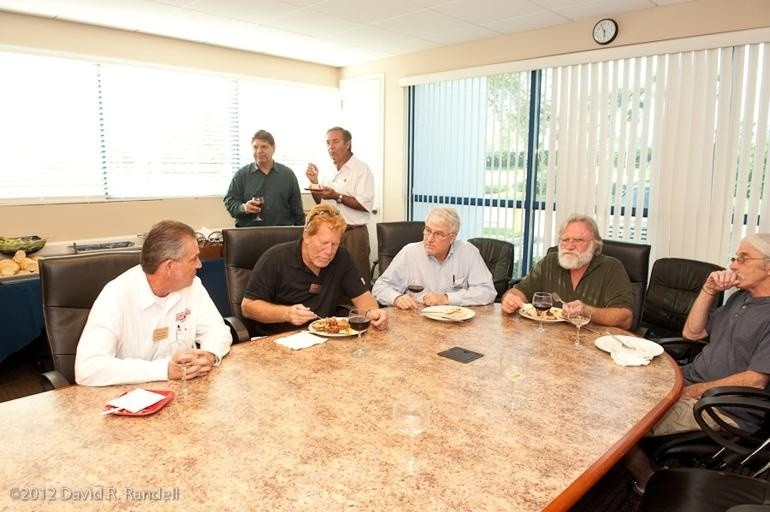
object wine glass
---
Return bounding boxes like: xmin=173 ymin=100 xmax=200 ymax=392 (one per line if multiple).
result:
xmin=407 ymin=273 xmax=426 ymax=314
xmin=532 ymin=292 xmax=552 ymax=333
xmin=166 ymin=339 xmax=199 ymax=392
xmin=349 ymin=308 xmax=371 ymax=357
xmin=566 ymin=302 xmax=594 ymax=348
xmin=251 ymin=195 xmax=265 ymax=222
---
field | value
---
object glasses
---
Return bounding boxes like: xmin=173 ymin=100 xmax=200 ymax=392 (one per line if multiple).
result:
xmin=306 ymin=209 xmax=339 ymax=226
xmin=732 ymin=256 xmax=766 ymax=264
xmin=423 ymin=227 xmax=452 ymax=238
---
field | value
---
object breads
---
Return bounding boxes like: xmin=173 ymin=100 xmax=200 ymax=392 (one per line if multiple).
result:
xmin=0 ymin=250 xmax=41 ymax=277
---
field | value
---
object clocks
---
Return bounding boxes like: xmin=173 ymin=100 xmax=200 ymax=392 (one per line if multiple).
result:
xmin=591 ymin=17 xmax=620 ymax=46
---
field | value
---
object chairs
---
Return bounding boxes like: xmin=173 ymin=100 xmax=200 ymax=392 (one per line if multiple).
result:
xmin=0 ymin=302 xmax=684 ymax=512
xmin=467 ymin=237 xmax=516 ymax=287
xmin=222 ymin=222 xmax=311 ymax=344
xmin=638 ymin=385 xmax=770 ymax=512
xmin=596 ymin=240 xmax=654 ymax=331
xmin=38 ymin=245 xmax=142 ymax=391
xmin=643 ymin=257 xmax=731 ymax=364
xmin=376 ymin=219 xmax=422 ymax=282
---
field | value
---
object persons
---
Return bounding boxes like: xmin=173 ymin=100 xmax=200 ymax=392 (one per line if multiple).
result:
xmin=370 ymin=206 xmax=498 ymax=313
xmin=220 ymin=130 xmax=307 ymax=227
xmin=240 ymin=203 xmax=390 ymax=337
xmin=304 ymin=125 xmax=377 ymax=295
xmin=73 ymin=220 xmax=234 ymax=389
xmin=622 ymin=228 xmax=770 ymax=512
xmin=501 ymin=214 xmax=638 ymax=332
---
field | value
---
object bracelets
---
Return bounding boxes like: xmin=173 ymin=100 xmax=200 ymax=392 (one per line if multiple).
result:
xmin=699 ymin=282 xmax=717 ymax=297
xmin=336 ymin=194 xmax=344 ymax=204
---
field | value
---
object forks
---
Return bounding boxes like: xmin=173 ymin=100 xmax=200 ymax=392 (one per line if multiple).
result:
xmin=606 ymin=330 xmax=635 ymax=349
xmin=551 ymin=291 xmax=569 ymax=306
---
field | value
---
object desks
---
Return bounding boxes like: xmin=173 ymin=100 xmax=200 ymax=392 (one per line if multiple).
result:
xmin=0 ymin=226 xmax=231 ymax=369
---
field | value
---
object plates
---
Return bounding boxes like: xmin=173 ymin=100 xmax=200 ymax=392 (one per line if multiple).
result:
xmin=0 ymin=233 xmax=46 ymax=256
xmin=304 ymin=189 xmax=326 ymax=192
xmin=309 ymin=316 xmax=364 ymax=337
xmin=594 ymin=335 xmax=665 ymax=357
xmin=518 ymin=305 xmax=568 ymax=322
xmin=107 ymin=388 xmax=175 ymax=417
xmin=420 ymin=304 xmax=475 ymax=324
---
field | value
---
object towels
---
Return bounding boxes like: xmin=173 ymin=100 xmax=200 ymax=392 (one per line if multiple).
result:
xmin=108 ymin=386 xmax=160 ymax=413
xmin=606 ymin=338 xmax=650 ymax=367
xmin=278 ymin=328 xmax=325 ymax=352
